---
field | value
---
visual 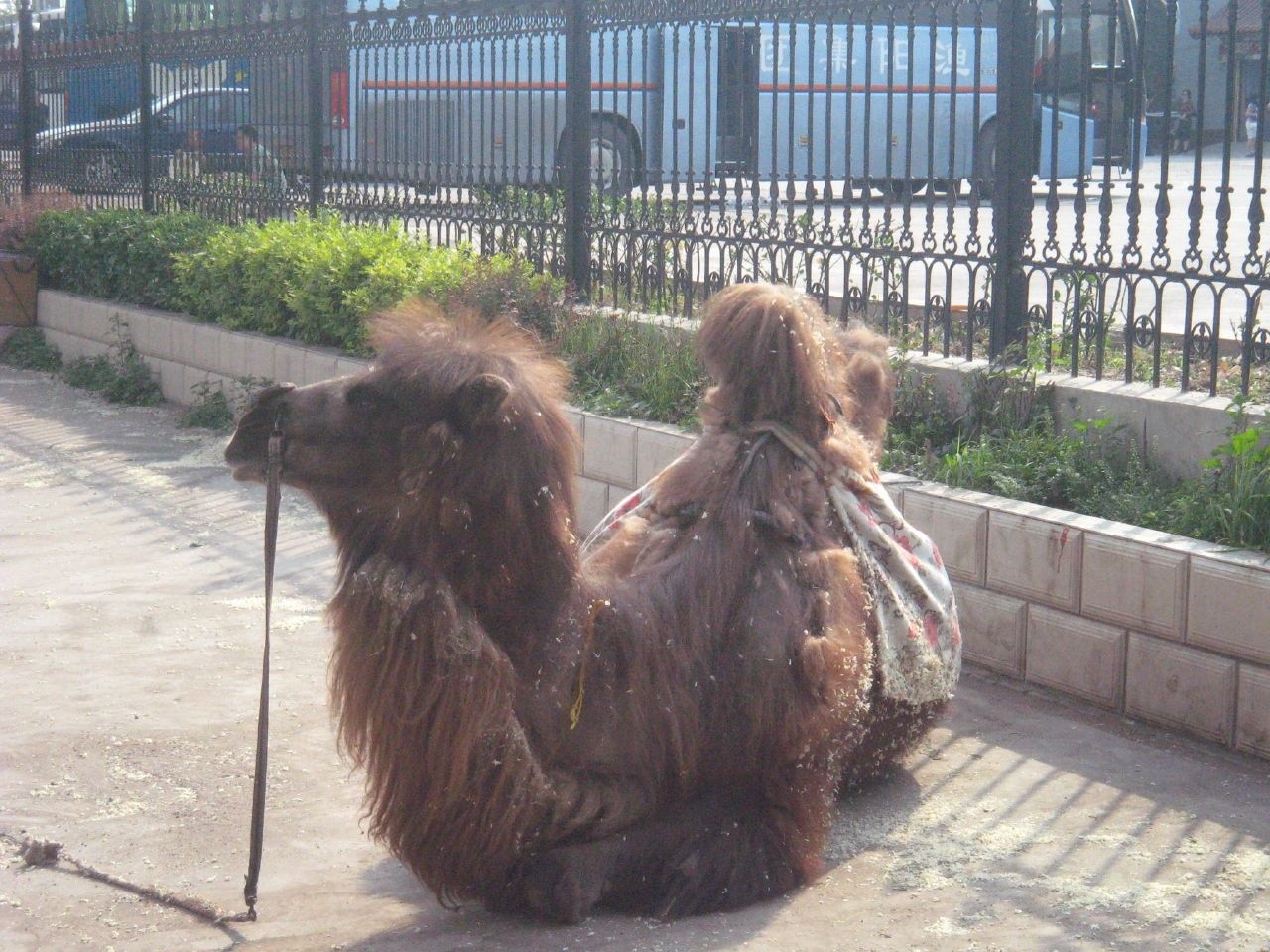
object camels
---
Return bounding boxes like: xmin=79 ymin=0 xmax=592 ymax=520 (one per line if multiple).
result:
xmin=223 ymin=275 xmax=962 ymax=918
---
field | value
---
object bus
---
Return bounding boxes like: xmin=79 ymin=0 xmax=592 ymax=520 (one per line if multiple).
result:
xmin=245 ymin=1 xmax=1149 ymax=208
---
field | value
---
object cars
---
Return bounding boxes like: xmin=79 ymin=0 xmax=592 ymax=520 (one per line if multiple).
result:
xmin=32 ymin=84 xmax=249 ymax=195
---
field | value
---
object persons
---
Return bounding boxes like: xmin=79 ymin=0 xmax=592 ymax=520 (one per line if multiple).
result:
xmin=1245 ymin=94 xmax=1259 ymax=156
xmin=35 ymin=90 xmax=49 ymax=133
xmin=1171 ymin=90 xmax=1193 ymax=153
xmin=238 ymin=124 xmax=287 ymax=197
